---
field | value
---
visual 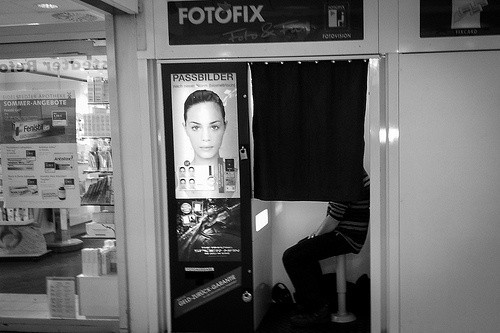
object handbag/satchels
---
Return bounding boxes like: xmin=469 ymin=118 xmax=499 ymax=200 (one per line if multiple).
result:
xmin=269 ymin=281 xmax=294 ymax=318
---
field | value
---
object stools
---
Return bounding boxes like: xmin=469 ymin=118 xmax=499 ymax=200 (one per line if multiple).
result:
xmin=329 ymin=253 xmax=358 ymax=323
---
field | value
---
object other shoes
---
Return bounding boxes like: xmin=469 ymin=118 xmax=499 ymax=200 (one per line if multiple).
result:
xmin=291 ymin=306 xmax=329 ymax=324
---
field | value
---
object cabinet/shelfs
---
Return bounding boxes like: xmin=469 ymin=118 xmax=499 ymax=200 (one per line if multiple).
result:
xmin=76 ymin=75 xmax=120 ymax=322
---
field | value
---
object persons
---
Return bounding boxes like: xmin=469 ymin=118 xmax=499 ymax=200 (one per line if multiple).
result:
xmin=282 ymin=141 xmax=371 ymax=333
xmin=174 ymin=90 xmax=240 ymax=199
